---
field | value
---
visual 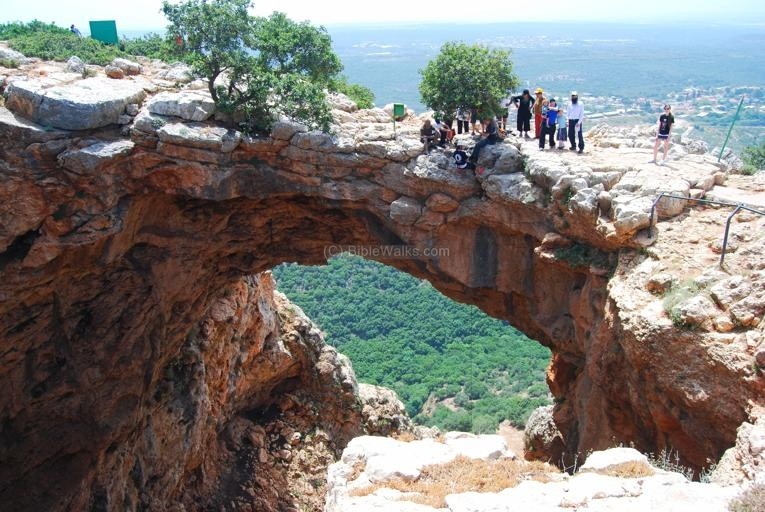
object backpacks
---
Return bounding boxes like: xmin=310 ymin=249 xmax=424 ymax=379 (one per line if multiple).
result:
xmin=446 ymin=129 xmax=455 ymax=140
xmin=497 ymin=128 xmax=505 ymax=142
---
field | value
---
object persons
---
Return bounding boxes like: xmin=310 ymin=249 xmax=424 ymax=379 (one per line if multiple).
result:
xmin=419 ymin=87 xmax=585 ymax=169
xmin=67 ymin=23 xmax=195 ymax=53
xmin=647 ymin=103 xmax=674 ymax=167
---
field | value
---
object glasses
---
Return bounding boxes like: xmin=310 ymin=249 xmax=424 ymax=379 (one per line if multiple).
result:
xmin=664 ymin=108 xmax=670 ymax=110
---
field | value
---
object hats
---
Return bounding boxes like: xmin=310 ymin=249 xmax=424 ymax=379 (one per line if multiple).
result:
xmin=542 ymin=100 xmax=549 ymax=105
xmin=572 ymin=90 xmax=578 ymax=96
xmin=534 ymin=87 xmax=543 ymax=94
xmin=549 ymin=98 xmax=555 ymax=102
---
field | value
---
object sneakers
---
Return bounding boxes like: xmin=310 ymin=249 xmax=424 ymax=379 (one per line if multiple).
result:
xmin=519 ymin=133 xmax=531 ymax=138
xmin=470 ymin=131 xmax=476 ymax=135
xmin=424 ymin=140 xmax=452 ymax=155
xmin=538 ymin=143 xmax=583 ymax=154
xmin=647 ymin=159 xmax=666 ymax=166
